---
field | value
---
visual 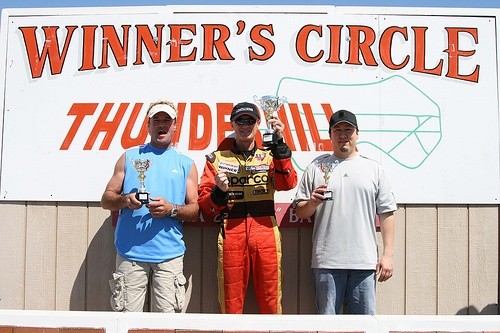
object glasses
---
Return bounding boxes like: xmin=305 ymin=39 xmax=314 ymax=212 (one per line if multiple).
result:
xmin=233 ymin=118 xmax=256 ymax=125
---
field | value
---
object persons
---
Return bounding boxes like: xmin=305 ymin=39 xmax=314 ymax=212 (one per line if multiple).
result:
xmin=197 ymin=102 xmax=298 ymax=314
xmin=100 ymin=101 xmax=200 ymax=313
xmin=292 ymin=110 xmax=397 ymax=315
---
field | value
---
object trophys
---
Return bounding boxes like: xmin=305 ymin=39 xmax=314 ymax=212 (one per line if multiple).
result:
xmin=319 ymin=162 xmax=335 ymax=201
xmin=132 ymin=157 xmax=150 ymax=204
xmin=253 ymin=94 xmax=287 ymax=147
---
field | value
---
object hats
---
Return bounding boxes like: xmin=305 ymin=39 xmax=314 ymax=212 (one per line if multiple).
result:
xmin=148 ymin=104 xmax=177 ymax=124
xmin=230 ymin=102 xmax=261 ymax=121
xmin=330 ymin=110 xmax=357 ymax=129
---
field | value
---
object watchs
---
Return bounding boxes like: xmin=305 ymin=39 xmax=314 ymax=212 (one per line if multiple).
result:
xmin=169 ymin=204 xmax=178 ymax=218
